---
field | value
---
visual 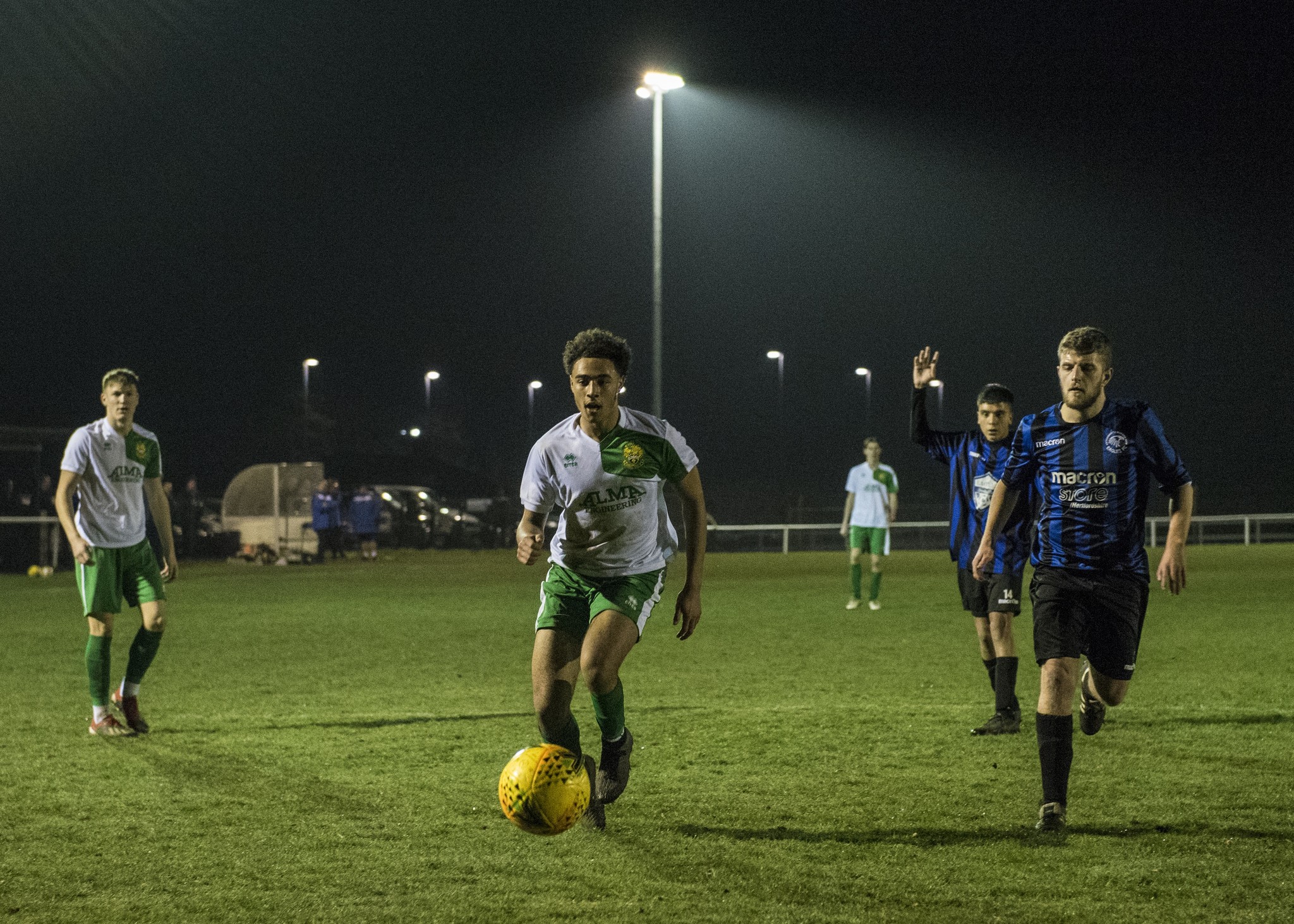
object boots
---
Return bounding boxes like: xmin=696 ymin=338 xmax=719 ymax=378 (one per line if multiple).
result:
xmin=981 ymin=657 xmax=1022 ymax=724
xmin=969 ymin=656 xmax=1020 ymax=736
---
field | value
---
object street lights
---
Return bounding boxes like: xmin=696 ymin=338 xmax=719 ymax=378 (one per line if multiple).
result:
xmin=634 ymin=68 xmax=685 ymax=420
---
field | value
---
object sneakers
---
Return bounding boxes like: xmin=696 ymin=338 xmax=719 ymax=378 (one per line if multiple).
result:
xmin=846 ymin=596 xmax=862 ymax=610
xmin=1035 ymin=802 xmax=1067 ymax=831
xmin=1079 ymin=660 xmax=1106 ymax=735
xmin=110 ymin=687 xmax=149 ymax=734
xmin=88 ymin=713 xmax=136 ymax=737
xmin=595 ymin=727 xmax=634 ymax=805
xmin=869 ymin=599 xmax=881 ymax=610
xmin=582 ymin=754 xmax=605 ymax=832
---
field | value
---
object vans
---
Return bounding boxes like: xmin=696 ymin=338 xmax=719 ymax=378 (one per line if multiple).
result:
xmin=351 ymin=483 xmax=565 ymax=552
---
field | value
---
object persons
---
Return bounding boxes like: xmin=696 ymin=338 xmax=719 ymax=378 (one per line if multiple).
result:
xmin=968 ymin=326 xmax=1200 ymax=834
xmin=838 ymin=436 xmax=901 ymax=611
xmin=911 ymin=343 xmax=1038 ymax=735
xmin=1 ymin=466 xmax=395 ymax=573
xmin=53 ymin=366 xmax=180 ymax=739
xmin=510 ymin=331 xmax=714 ymax=810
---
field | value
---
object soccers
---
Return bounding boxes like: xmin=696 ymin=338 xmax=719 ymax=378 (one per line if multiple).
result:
xmin=497 ymin=742 xmax=590 ymax=837
